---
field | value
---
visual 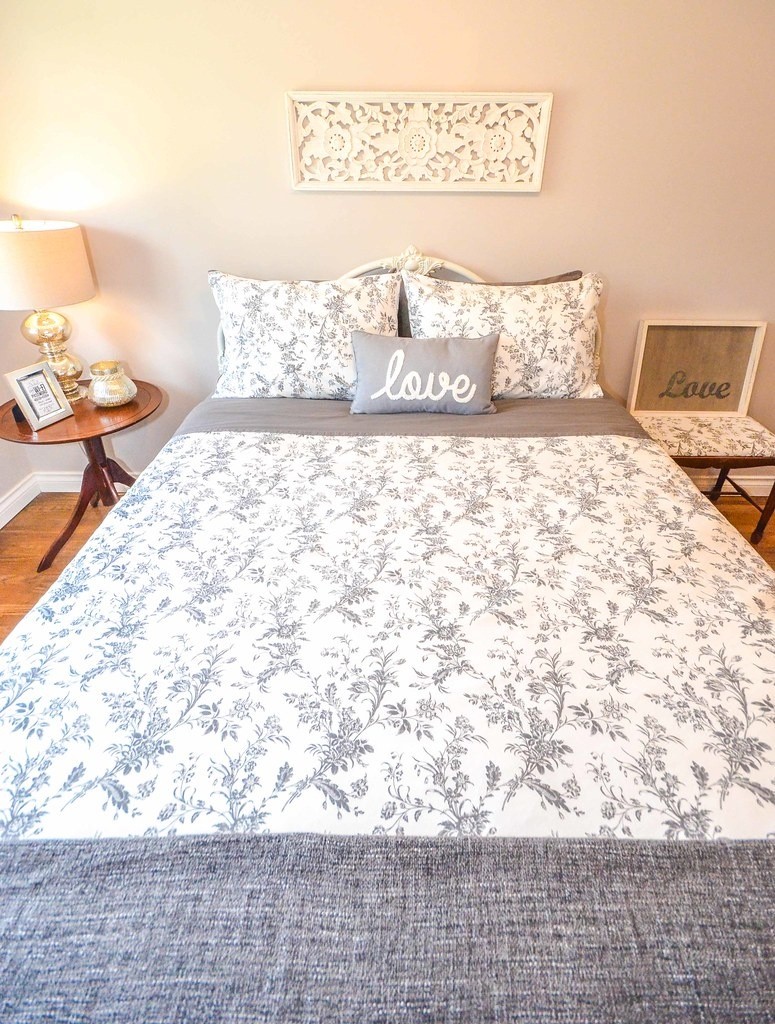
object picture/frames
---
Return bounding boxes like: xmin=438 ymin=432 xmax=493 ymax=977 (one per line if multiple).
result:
xmin=4 ymin=361 xmax=74 ymax=433
xmin=623 ymin=319 xmax=766 ymax=418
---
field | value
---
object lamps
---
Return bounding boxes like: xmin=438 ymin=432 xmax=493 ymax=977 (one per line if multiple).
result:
xmin=0 ymin=217 xmax=96 ymax=403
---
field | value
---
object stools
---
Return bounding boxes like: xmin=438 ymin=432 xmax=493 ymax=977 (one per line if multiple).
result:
xmin=631 ymin=415 xmax=775 ymax=544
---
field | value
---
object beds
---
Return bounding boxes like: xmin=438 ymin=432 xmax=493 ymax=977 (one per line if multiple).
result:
xmin=0 ymin=245 xmax=775 ymax=1024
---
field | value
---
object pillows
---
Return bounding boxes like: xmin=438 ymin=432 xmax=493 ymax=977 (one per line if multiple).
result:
xmin=401 ymin=266 xmax=605 ymax=400
xmin=349 ymin=330 xmax=500 ymax=415
xmin=206 ymin=263 xmax=401 ymax=402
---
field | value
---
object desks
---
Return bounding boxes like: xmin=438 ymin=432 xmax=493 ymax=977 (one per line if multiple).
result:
xmin=0 ymin=379 xmax=163 ymax=573
